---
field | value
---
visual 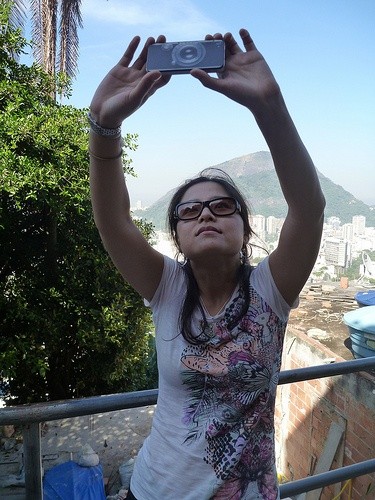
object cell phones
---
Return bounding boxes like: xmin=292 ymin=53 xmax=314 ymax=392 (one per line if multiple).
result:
xmin=146 ymin=39 xmax=226 ymax=74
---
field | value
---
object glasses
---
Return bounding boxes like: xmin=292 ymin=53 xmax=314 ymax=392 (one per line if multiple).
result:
xmin=174 ymin=196 xmax=241 ymax=220
